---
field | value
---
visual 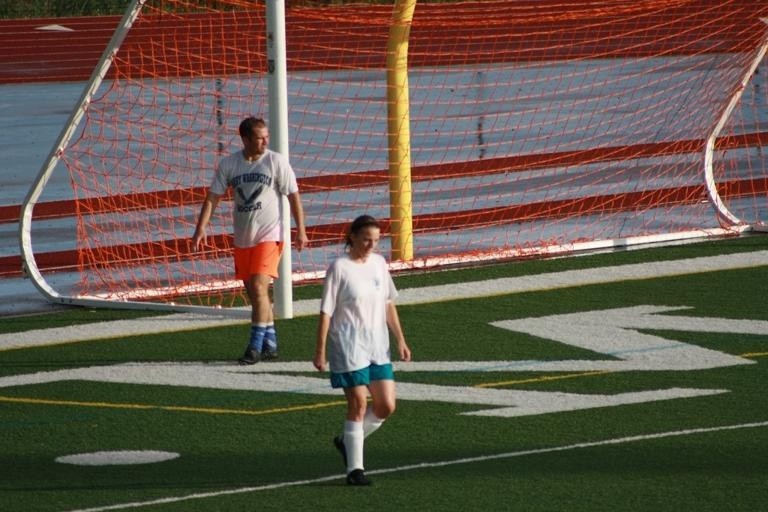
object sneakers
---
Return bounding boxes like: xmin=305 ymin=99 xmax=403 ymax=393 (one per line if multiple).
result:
xmin=333 ymin=437 xmax=371 ymax=485
xmin=238 ymin=345 xmax=278 ymax=366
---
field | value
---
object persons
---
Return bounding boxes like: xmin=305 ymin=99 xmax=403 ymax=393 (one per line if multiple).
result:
xmin=310 ymin=213 xmax=412 ymax=486
xmin=188 ymin=116 xmax=310 ymax=367
